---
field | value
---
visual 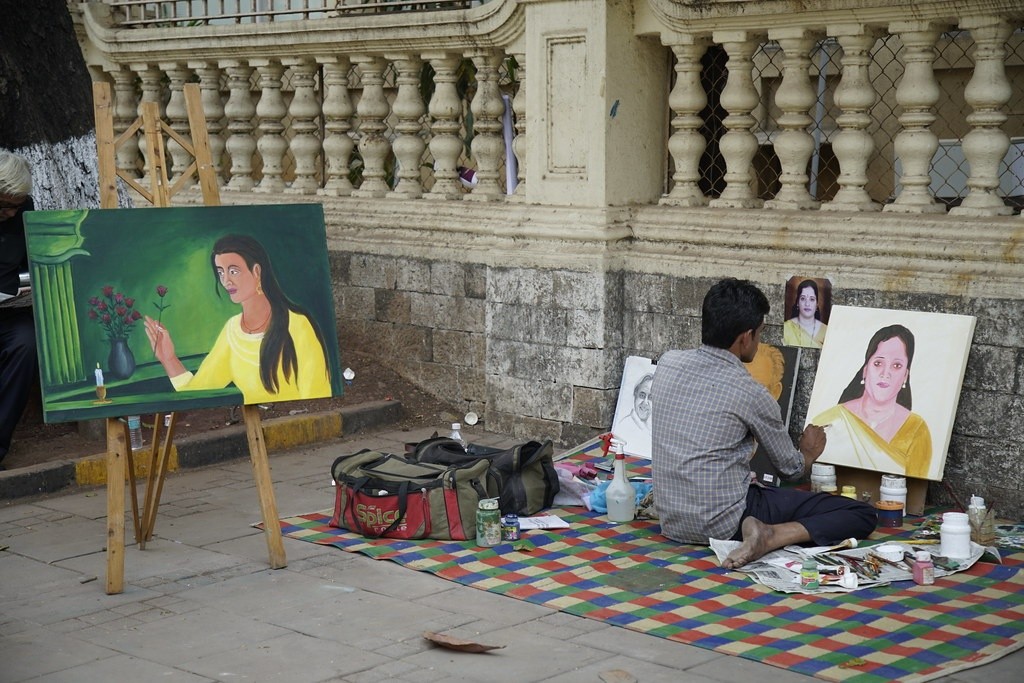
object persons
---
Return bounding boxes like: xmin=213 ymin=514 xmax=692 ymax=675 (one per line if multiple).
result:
xmin=653 ymin=278 xmax=877 ymax=570
xmin=0 ymin=152 xmax=37 ymax=463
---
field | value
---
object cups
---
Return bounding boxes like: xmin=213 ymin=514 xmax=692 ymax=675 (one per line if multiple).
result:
xmin=966 ymin=508 xmax=995 ymax=547
xmin=464 ymin=412 xmax=478 ymax=425
xmin=862 ymin=490 xmax=872 ymax=504
xmin=875 ymin=500 xmax=905 ymax=528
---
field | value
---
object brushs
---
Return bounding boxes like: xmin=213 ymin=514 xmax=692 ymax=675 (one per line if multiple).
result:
xmin=912 ymin=547 xmax=960 ymax=569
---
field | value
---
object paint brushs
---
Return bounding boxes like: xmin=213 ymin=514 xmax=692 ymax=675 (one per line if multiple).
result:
xmin=947 ymin=485 xmax=978 ymax=532
xmin=903 ymin=556 xmax=952 ymax=571
xmin=980 ymin=501 xmax=995 ymax=528
xmin=800 ymin=424 xmax=832 ymax=435
xmin=812 ymin=553 xmax=883 ymax=581
xmin=868 ymin=553 xmax=908 ymax=571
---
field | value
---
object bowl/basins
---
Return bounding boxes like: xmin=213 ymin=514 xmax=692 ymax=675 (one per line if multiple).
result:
xmin=875 ymin=545 xmax=905 ymax=563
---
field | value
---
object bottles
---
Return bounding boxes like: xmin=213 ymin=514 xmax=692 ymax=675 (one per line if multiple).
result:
xmin=821 ymin=485 xmax=837 ymax=495
xmin=504 ymin=515 xmax=520 ymax=541
xmin=449 ymin=423 xmax=468 ymax=453
xmin=801 ymin=560 xmax=819 ymax=590
xmin=810 ymin=463 xmax=836 ymax=492
xmin=475 ymin=498 xmax=502 ymax=547
xmin=880 ymin=474 xmax=907 ymax=519
xmin=913 ymin=551 xmax=936 ymax=585
xmin=969 ymin=496 xmax=985 ymax=510
xmin=940 ymin=512 xmax=972 ymax=560
xmin=840 ymin=486 xmax=857 ymax=501
xmin=128 ymin=415 xmax=143 ymax=450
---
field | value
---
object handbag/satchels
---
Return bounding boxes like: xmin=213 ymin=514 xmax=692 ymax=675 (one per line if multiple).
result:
xmin=330 ymin=448 xmax=489 ymax=541
xmin=406 ymin=430 xmax=560 ymax=517
